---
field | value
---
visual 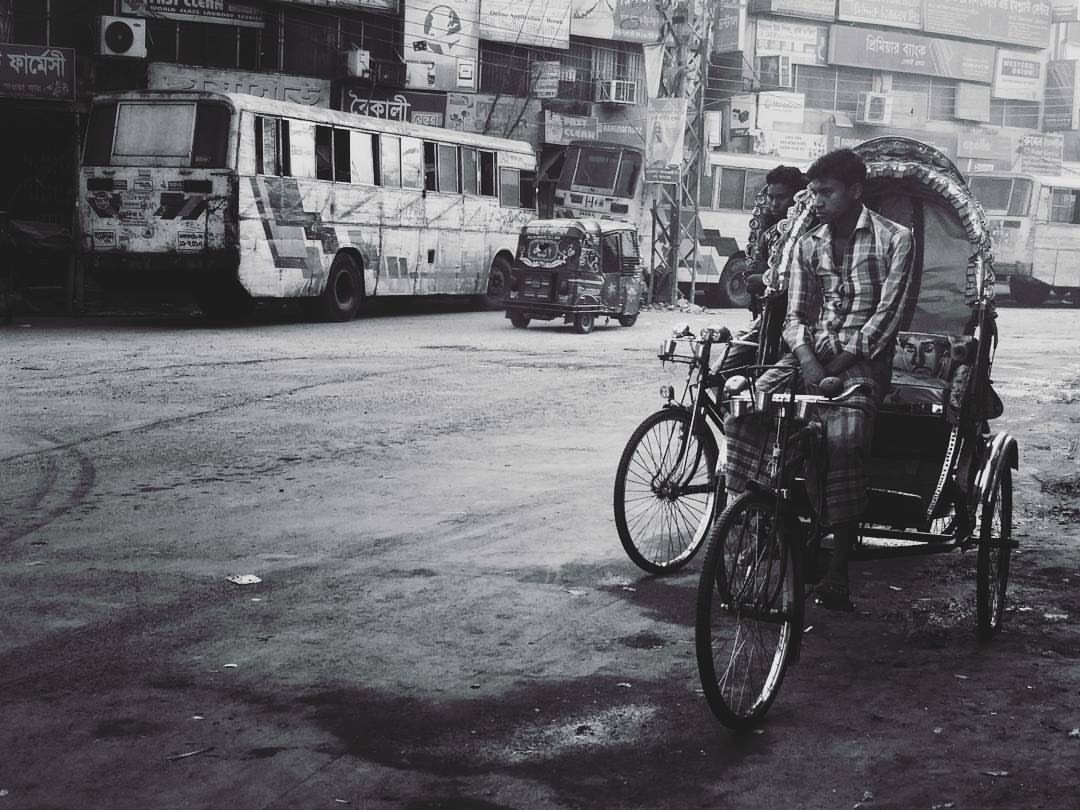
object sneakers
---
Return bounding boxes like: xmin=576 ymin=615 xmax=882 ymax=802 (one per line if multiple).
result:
xmin=820 ymin=555 xmax=852 ymax=612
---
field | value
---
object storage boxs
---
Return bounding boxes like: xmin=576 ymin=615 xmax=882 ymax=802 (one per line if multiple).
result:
xmin=593 ymin=79 xmax=638 ymax=105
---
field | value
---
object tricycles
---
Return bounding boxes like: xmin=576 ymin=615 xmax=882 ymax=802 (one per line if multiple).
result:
xmin=613 ymin=135 xmax=1019 ymax=734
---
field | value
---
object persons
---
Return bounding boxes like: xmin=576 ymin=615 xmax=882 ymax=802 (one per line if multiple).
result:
xmin=715 ymin=167 xmax=802 ymax=382
xmin=724 ymin=148 xmax=918 ymax=611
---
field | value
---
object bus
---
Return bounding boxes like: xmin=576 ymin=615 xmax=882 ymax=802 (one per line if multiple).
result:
xmin=71 ymin=88 xmax=824 ymax=334
xmin=961 ymin=170 xmax=1080 ymax=308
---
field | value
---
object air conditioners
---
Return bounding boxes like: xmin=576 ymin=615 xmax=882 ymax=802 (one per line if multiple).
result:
xmin=93 ymin=14 xmax=147 ymax=59
xmin=335 ymin=50 xmax=370 ymax=79
xmin=855 ymin=91 xmax=895 ymax=125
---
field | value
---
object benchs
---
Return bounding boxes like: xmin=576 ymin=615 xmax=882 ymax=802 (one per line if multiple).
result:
xmin=876 ymin=326 xmax=974 ymax=417
